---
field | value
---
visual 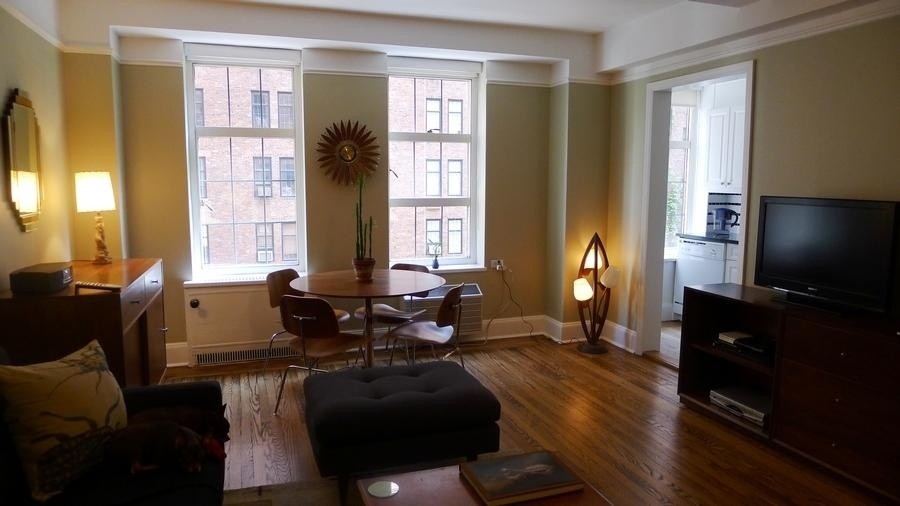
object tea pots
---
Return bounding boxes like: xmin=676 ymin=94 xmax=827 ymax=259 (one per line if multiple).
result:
xmin=711 ymin=207 xmax=738 ymax=235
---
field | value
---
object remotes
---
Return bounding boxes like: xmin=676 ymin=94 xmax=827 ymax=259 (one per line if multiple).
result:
xmin=75 ymin=281 xmax=121 ymax=291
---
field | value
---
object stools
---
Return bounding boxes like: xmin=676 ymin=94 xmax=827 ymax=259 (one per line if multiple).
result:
xmin=302 ymin=360 xmax=501 ymax=503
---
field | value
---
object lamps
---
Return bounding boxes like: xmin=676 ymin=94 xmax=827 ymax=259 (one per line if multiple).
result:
xmin=573 ymin=231 xmax=620 ymax=355
xmin=74 ymin=171 xmax=116 ymax=266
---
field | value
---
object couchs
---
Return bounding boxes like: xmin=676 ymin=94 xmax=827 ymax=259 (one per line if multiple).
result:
xmin=1 ymin=345 xmax=226 ymax=505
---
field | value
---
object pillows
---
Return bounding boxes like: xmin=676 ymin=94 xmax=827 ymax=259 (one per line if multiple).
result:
xmin=1 ymin=339 xmax=130 ymax=501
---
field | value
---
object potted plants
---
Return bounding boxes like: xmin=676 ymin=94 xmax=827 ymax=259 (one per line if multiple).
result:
xmin=427 ymin=239 xmax=442 ymax=270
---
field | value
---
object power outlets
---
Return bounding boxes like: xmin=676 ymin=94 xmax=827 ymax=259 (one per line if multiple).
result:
xmin=491 ymin=260 xmax=503 ymax=268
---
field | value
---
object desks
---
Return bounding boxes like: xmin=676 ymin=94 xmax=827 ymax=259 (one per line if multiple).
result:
xmin=288 ymin=268 xmax=445 ymax=368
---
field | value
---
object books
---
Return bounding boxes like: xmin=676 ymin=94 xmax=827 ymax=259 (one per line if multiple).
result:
xmin=458 ymin=451 xmax=589 ymax=506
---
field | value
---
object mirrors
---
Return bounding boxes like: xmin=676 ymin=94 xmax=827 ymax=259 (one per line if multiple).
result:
xmin=3 ymin=88 xmax=44 ymax=234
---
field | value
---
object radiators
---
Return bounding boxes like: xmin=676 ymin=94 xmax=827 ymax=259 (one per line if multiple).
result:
xmin=185 ymin=288 xmax=304 ymax=366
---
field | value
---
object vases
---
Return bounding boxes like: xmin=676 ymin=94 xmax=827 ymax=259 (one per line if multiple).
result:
xmin=350 ymin=256 xmax=378 ymax=281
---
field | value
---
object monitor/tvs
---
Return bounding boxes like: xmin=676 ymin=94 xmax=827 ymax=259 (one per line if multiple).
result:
xmin=754 ymin=195 xmax=900 ymax=323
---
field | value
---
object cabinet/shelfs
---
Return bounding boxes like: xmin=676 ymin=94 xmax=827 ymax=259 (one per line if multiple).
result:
xmin=676 ymin=282 xmax=900 ymax=505
xmin=671 ymin=230 xmax=740 ymax=320
xmin=1 ymin=246 xmax=168 ymax=389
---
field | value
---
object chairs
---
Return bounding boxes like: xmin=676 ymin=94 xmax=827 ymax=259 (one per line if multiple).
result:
xmin=385 ymin=283 xmax=467 ymax=369
xmin=272 ymin=294 xmax=377 ymax=416
xmin=355 ymin=264 xmax=428 ymax=369
xmin=261 ymin=269 xmax=351 ymax=377
